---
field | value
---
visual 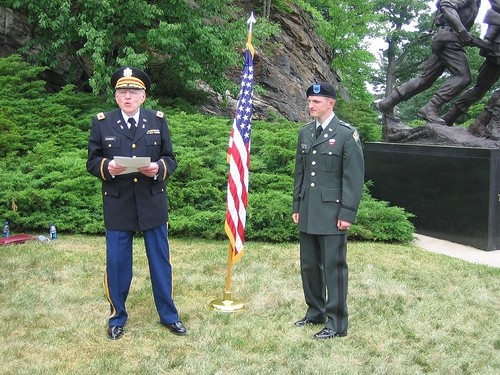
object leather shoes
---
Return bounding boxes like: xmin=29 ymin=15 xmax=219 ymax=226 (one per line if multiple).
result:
xmin=294 ymin=317 xmax=326 ymax=325
xmin=107 ymin=325 xmax=124 ymax=340
xmin=314 ymin=327 xmax=347 ymax=338
xmin=168 ymin=321 xmax=186 ymax=334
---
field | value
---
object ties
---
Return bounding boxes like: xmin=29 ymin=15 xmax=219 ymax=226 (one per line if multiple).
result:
xmin=316 ymin=125 xmax=323 ymax=138
xmin=128 ymin=118 xmax=137 ymax=134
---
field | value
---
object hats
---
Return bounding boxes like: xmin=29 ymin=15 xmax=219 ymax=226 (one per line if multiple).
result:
xmin=306 ymin=82 xmax=336 ymax=99
xmin=111 ymin=67 xmax=151 ymax=92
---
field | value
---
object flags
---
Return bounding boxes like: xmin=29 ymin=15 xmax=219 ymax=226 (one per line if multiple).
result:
xmin=224 ymin=32 xmax=255 ymax=264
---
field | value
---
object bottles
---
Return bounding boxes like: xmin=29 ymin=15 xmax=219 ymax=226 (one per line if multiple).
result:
xmin=2 ymin=221 xmax=10 ymax=238
xmin=50 ymin=225 xmax=57 ymax=240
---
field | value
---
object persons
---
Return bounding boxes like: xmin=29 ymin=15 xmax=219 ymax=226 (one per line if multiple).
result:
xmin=378 ymin=0 xmax=500 ymax=141
xmin=292 ymin=83 xmax=364 ymax=340
xmin=86 ymin=67 xmax=187 ymax=340
